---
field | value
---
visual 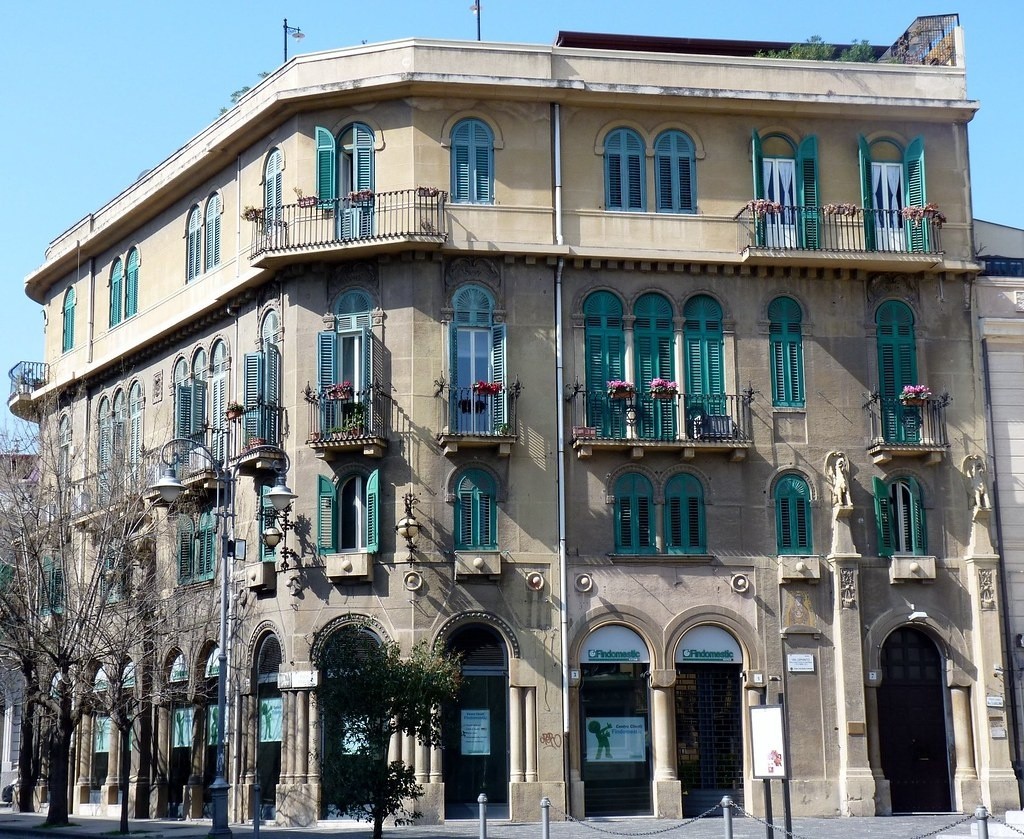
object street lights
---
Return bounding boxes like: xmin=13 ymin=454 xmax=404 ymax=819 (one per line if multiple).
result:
xmin=470 ymin=0 xmax=481 ymax=40
xmin=281 ymin=18 xmax=306 ymax=66
xmin=141 ymin=436 xmax=303 ymax=838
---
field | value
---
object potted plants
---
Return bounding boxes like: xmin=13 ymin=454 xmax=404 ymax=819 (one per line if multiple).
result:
xmin=900 ymin=386 xmax=929 ymax=408
xmin=227 ymin=403 xmax=242 ymax=420
xmin=331 ymin=424 xmax=359 ymax=441
xmin=476 ymin=380 xmax=501 ymax=396
xmin=607 ymin=380 xmax=637 ymax=400
xmin=326 ymin=380 xmax=351 ymax=399
xmin=649 ymin=377 xmax=677 ymax=400
